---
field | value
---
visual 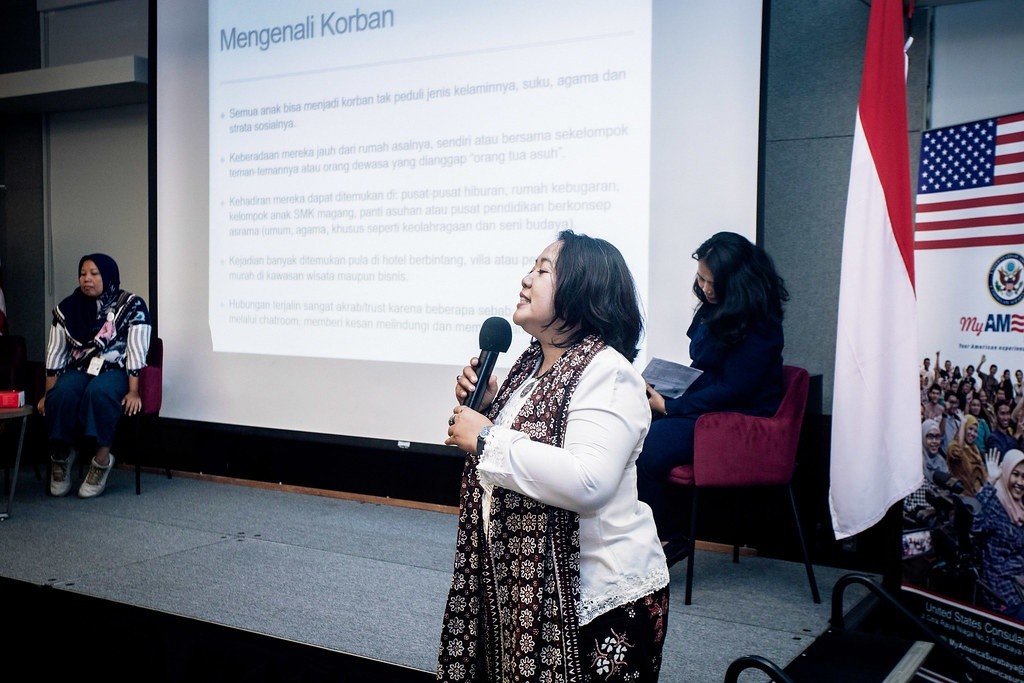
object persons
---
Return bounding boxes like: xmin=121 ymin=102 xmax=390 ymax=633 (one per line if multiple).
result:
xmin=903 ymin=351 xmax=1024 ymax=621
xmin=37 ymin=253 xmax=151 ymax=498
xmin=436 ymin=229 xmax=669 ymax=683
xmin=636 ymin=230 xmax=790 ymax=569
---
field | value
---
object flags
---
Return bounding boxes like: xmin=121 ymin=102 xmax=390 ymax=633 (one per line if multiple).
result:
xmin=826 ymin=0 xmax=926 ymax=542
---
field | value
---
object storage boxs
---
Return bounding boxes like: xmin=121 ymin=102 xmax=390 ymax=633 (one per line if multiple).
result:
xmin=0 ymin=389 xmax=25 ymax=408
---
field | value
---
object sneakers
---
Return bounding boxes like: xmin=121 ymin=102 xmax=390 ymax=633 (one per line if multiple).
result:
xmin=50 ymin=448 xmax=76 ymax=496
xmin=78 ymin=453 xmax=115 ymax=498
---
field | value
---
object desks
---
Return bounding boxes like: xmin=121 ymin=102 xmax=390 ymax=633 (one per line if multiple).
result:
xmin=0 ymin=404 xmax=32 ymax=521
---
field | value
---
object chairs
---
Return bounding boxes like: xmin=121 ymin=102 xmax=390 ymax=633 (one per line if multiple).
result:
xmin=671 ymin=366 xmax=821 ymax=605
xmin=723 ymin=574 xmax=1013 ymax=683
xmin=33 ymin=336 xmax=172 ymax=495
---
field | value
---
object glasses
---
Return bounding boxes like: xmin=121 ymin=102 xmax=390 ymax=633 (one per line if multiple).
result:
xmin=949 ymin=400 xmax=957 ymax=404
xmin=926 ymin=432 xmax=942 ymax=442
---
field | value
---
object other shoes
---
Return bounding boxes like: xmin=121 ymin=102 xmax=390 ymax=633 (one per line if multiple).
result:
xmin=662 ymin=535 xmax=689 ymax=568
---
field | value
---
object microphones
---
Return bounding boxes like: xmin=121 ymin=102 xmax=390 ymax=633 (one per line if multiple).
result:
xmin=462 ymin=317 xmax=513 ymax=412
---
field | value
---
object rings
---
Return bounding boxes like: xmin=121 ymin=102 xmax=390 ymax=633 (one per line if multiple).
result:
xmin=457 ymin=375 xmax=462 ymax=382
xmin=449 ymin=414 xmax=457 ymax=426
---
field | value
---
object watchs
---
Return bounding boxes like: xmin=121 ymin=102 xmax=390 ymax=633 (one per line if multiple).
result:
xmin=476 ymin=425 xmax=493 ymax=460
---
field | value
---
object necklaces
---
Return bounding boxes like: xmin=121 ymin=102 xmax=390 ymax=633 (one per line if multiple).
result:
xmin=520 ymin=354 xmax=550 ymax=399
